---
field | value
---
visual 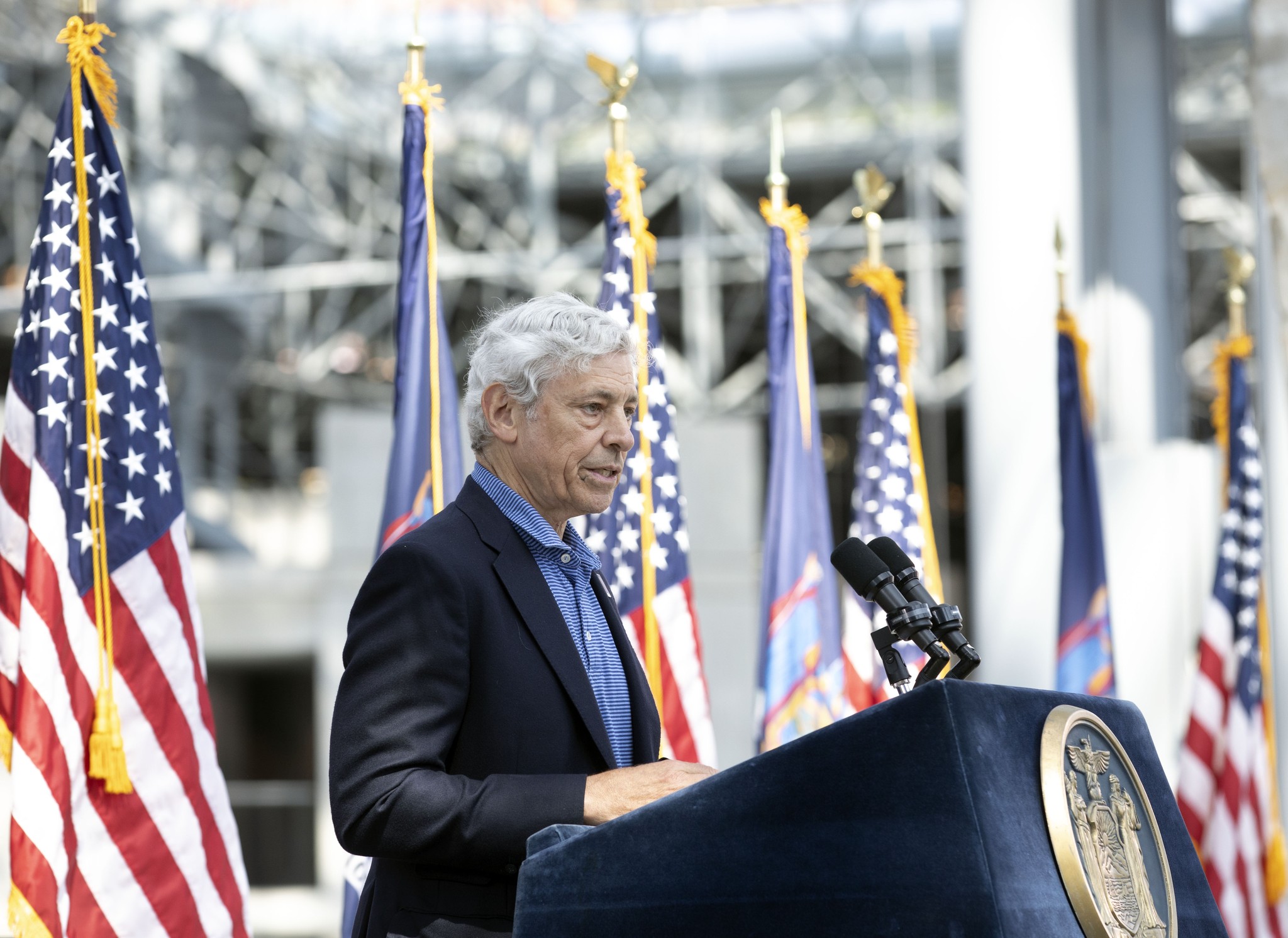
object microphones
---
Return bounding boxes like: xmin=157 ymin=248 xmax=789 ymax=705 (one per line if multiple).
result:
xmin=831 ymin=536 xmax=981 ymax=690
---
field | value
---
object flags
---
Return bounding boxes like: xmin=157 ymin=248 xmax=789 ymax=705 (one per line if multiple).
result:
xmin=0 ymin=15 xmax=250 ymax=938
xmin=344 ymin=91 xmax=1288 ymax=938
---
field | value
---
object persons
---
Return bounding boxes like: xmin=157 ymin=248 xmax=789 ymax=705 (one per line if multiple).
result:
xmin=330 ymin=294 xmax=721 ymax=938
xmin=1109 ymin=774 xmax=1167 ymax=930
xmin=1064 ymin=770 xmax=1120 ymax=928
